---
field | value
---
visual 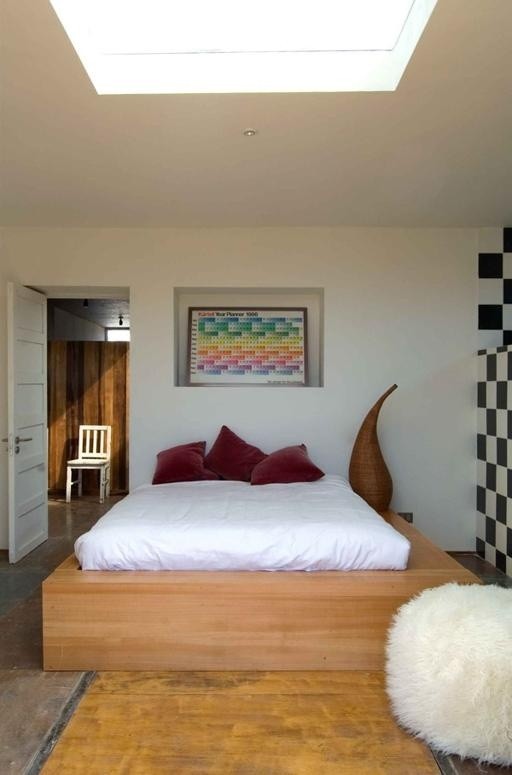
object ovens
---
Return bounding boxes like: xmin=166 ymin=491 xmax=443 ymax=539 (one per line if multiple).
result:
xmin=40 ymin=472 xmax=482 ymax=672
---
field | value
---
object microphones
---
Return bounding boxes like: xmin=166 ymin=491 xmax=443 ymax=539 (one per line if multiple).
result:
xmin=382 ymin=582 xmax=512 ymax=767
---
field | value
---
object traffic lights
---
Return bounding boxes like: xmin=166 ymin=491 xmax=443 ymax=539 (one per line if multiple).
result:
xmin=151 ymin=440 xmax=206 ymax=484
xmin=251 ymin=442 xmax=326 ymax=485
xmin=202 ymin=466 xmax=219 ymax=479
xmin=203 ymin=424 xmax=269 ymax=482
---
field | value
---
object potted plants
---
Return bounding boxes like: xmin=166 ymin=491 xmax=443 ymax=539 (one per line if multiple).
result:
xmin=187 ymin=305 xmax=308 ymax=387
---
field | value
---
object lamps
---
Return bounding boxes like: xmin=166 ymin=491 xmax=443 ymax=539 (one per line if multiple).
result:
xmin=65 ymin=423 xmax=111 ymax=503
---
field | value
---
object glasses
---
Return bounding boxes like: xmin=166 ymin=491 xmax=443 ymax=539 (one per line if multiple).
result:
xmin=118 ymin=316 xmax=124 ymax=325
xmin=83 ymin=299 xmax=89 ymax=308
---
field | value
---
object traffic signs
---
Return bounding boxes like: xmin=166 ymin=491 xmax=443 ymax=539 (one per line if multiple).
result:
xmin=396 ymin=512 xmax=413 ymax=524
